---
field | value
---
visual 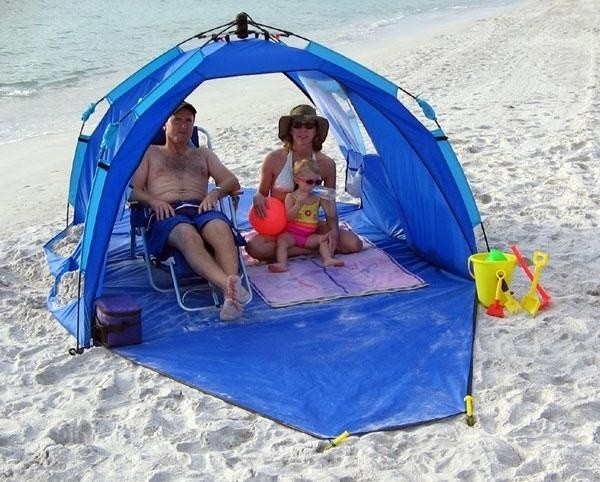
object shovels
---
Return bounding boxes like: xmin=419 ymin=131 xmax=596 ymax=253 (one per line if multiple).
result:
xmin=486 ymin=269 xmax=507 ymax=318
xmin=521 ymin=251 xmax=548 ymax=317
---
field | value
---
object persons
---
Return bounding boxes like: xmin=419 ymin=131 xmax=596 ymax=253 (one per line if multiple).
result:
xmin=131 ymin=102 xmax=250 ymax=322
xmin=267 ymin=158 xmax=344 ymax=273
xmin=245 ymin=104 xmax=363 ymax=262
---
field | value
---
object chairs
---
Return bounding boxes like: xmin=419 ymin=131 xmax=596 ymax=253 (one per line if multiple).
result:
xmin=128 ymin=124 xmax=252 ymax=311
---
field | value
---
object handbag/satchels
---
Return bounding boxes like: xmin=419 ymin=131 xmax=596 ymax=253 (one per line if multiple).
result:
xmin=94 ymin=292 xmax=143 ymax=348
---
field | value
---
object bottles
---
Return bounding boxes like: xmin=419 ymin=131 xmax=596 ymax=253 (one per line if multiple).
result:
xmin=311 ymin=184 xmax=339 ymax=204
xmin=317 ymin=207 xmax=326 ymax=222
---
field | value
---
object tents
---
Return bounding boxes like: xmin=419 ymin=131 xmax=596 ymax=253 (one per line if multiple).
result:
xmin=41 ymin=10 xmax=492 ymax=441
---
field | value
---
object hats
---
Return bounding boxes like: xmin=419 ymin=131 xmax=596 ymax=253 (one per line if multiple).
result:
xmin=173 ymin=101 xmax=196 ymax=115
xmin=278 ymin=104 xmax=329 ymax=146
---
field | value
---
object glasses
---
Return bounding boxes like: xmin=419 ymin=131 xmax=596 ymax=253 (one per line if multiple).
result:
xmin=291 ymin=121 xmax=316 ymax=129
xmin=297 ymin=176 xmax=323 ymax=186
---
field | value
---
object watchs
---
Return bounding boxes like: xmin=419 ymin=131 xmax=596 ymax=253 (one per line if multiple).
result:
xmin=211 ymin=186 xmax=225 ymax=197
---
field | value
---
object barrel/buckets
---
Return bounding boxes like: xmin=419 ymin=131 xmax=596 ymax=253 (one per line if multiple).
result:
xmin=468 ymin=252 xmax=516 ymax=306
